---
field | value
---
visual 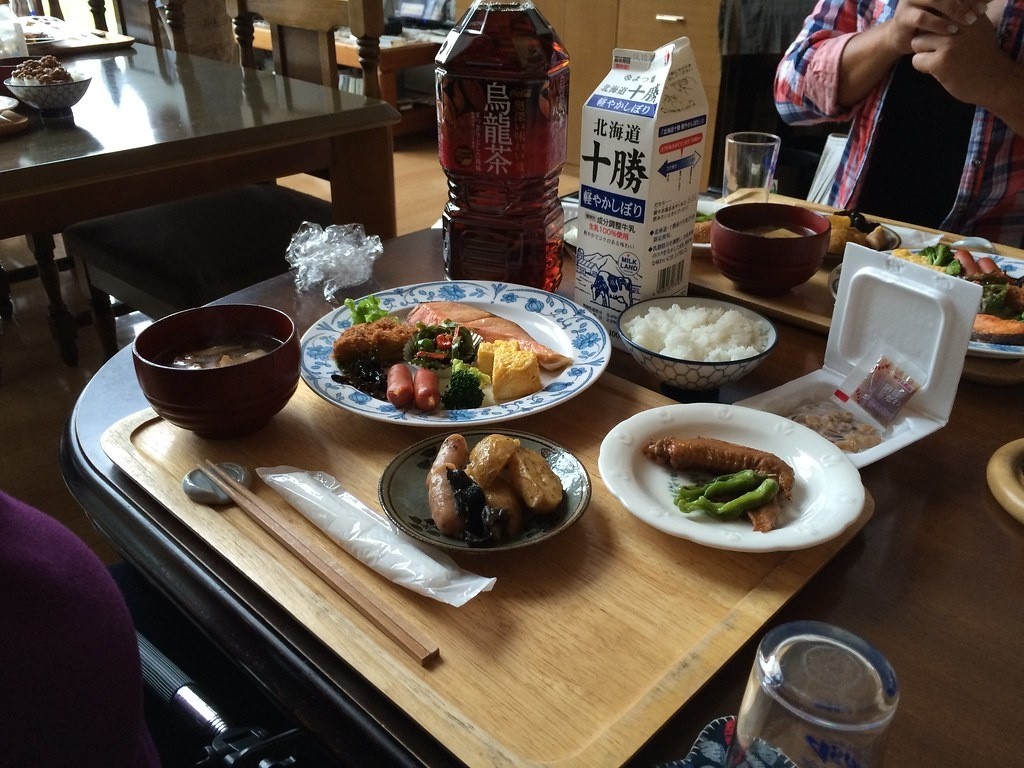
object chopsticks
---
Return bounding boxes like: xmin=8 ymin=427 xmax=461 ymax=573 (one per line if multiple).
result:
xmin=194 ymin=460 xmax=440 ymax=667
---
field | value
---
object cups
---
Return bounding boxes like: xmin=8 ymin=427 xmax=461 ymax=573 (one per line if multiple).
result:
xmin=723 ymin=130 xmax=781 ymax=204
xmin=739 ymin=622 xmax=898 ymax=768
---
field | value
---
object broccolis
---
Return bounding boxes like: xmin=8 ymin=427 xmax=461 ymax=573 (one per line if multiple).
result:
xmin=442 ymin=360 xmax=490 ymax=409
xmin=921 ymin=244 xmax=962 ymax=275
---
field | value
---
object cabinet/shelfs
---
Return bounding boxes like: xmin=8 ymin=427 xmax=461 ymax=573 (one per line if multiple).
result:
xmin=455 ymin=0 xmax=724 ymax=193
xmin=232 ymin=20 xmax=442 ymax=151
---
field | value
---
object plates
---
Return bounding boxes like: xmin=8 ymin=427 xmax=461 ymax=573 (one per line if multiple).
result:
xmin=597 ymin=403 xmax=865 ymax=553
xmin=376 ymin=431 xmax=591 ymax=552
xmin=300 ymin=281 xmax=612 ymax=427
xmin=818 ymin=211 xmax=901 ymax=264
xmin=830 ymin=252 xmax=1023 ymax=355
xmin=0 ymin=96 xmax=18 ymax=113
xmin=692 ymin=200 xmax=728 ymax=248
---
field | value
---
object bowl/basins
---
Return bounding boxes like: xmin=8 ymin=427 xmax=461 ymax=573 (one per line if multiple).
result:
xmin=712 ymin=202 xmax=832 ymax=291
xmin=562 ymin=217 xmax=577 ymax=260
xmin=734 ymin=240 xmax=985 ymax=464
xmin=132 ymin=304 xmax=302 ymax=436
xmin=619 ymin=297 xmax=776 ymax=386
xmin=4 ymin=73 xmax=92 ymax=111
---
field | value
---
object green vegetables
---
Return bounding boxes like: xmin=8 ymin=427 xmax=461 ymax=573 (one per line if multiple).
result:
xmin=344 ymin=295 xmax=401 ymax=324
xmin=696 ymin=211 xmax=716 ymax=222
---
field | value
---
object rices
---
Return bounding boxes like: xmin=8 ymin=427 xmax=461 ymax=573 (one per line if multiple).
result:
xmin=623 ymin=303 xmax=769 ymax=361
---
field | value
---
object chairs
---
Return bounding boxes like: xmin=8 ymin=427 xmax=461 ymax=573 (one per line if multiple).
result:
xmin=1 ymin=0 xmax=385 ymax=369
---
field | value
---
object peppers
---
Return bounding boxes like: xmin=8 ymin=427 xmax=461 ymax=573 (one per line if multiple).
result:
xmin=673 ymin=469 xmax=780 ymax=521
xmin=409 ymin=317 xmax=473 ymax=371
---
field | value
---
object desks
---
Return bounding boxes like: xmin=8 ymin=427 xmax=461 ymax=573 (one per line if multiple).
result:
xmin=59 ymin=182 xmax=1024 ymax=768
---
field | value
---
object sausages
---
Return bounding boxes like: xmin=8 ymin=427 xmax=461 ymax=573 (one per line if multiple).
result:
xmin=385 ymin=364 xmax=441 ymax=410
xmin=954 ymin=250 xmax=1004 ymax=277
xmin=425 ymin=434 xmax=470 ymax=537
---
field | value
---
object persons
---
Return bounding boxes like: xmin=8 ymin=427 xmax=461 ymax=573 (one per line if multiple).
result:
xmin=0 ymin=496 xmax=162 ymax=768
xmin=773 ymin=0 xmax=1024 ymax=249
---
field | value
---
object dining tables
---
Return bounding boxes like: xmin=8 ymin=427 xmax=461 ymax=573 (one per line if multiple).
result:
xmin=0 ymin=42 xmax=402 ymax=244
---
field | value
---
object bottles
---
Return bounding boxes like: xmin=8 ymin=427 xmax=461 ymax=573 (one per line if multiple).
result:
xmin=0 ymin=0 xmax=29 ymax=57
xmin=434 ymin=0 xmax=569 ymax=293
